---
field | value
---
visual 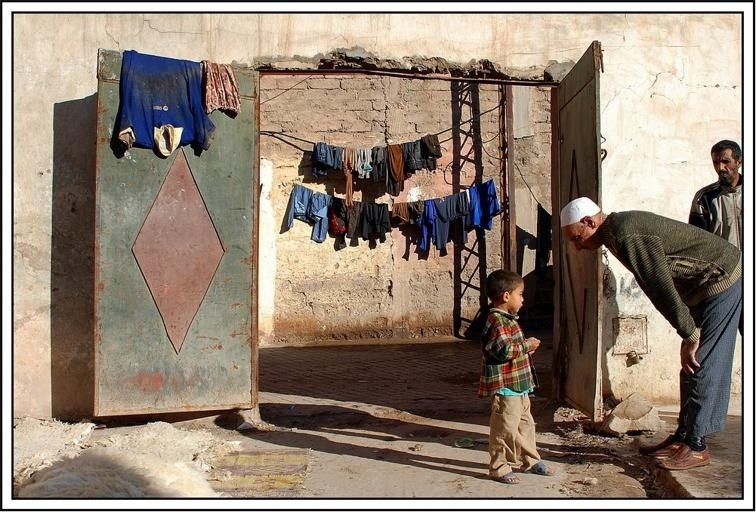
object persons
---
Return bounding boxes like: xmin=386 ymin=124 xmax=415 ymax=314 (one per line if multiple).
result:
xmin=559 ymin=196 xmax=743 ymax=472
xmin=478 ymin=268 xmax=554 ymax=484
xmin=687 ymin=139 xmax=743 ymax=340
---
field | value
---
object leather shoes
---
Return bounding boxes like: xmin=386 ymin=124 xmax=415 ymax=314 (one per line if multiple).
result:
xmin=639 ymin=434 xmax=683 ymax=456
xmin=655 ymin=446 xmax=711 ymax=469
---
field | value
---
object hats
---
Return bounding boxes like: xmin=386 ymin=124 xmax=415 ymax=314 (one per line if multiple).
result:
xmin=560 ymin=197 xmax=600 ymax=227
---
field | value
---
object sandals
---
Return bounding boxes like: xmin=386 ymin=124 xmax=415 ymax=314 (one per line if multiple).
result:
xmin=497 ymin=471 xmax=520 ymax=485
xmin=531 ymin=462 xmax=554 ymax=476
